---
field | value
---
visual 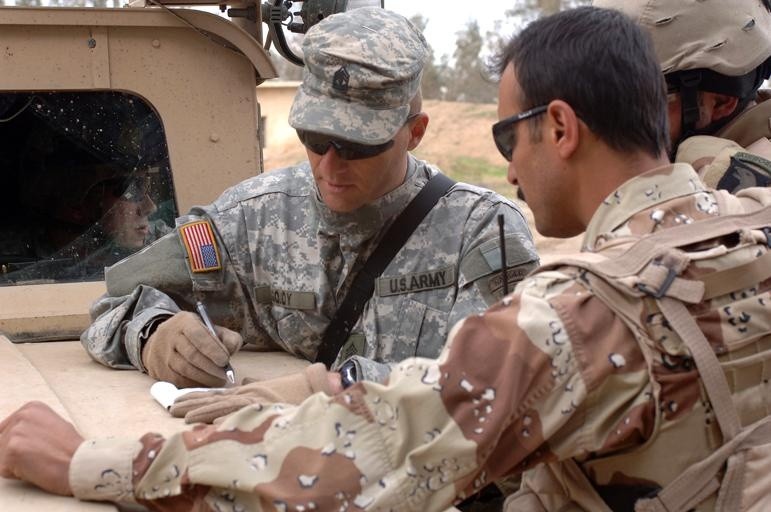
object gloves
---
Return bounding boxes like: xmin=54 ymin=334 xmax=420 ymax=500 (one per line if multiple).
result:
xmin=169 ymin=361 xmax=338 ymax=425
xmin=143 ymin=310 xmax=242 ymax=389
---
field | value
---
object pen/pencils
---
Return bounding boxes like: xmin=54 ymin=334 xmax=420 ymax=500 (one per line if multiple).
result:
xmin=197 ymin=301 xmax=235 ymax=384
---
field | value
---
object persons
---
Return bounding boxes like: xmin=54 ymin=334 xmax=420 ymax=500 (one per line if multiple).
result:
xmin=98 ymin=161 xmax=160 ymax=250
xmin=1 ymin=5 xmax=771 ymax=511
xmin=586 ymin=0 xmax=771 ymax=197
xmin=79 ymin=5 xmax=543 ymax=437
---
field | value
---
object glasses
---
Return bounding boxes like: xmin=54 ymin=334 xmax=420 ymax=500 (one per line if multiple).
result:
xmin=295 ymin=127 xmax=392 ymax=160
xmin=111 ymin=176 xmax=151 ymax=203
xmin=492 ymin=104 xmax=591 ymax=160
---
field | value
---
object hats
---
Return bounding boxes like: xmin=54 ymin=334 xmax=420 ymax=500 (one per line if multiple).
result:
xmin=287 ymin=7 xmax=425 ymax=146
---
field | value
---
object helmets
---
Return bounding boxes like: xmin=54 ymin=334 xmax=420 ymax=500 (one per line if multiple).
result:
xmin=592 ymin=0 xmax=771 ymax=98
xmin=69 ymin=140 xmax=149 ymax=211
